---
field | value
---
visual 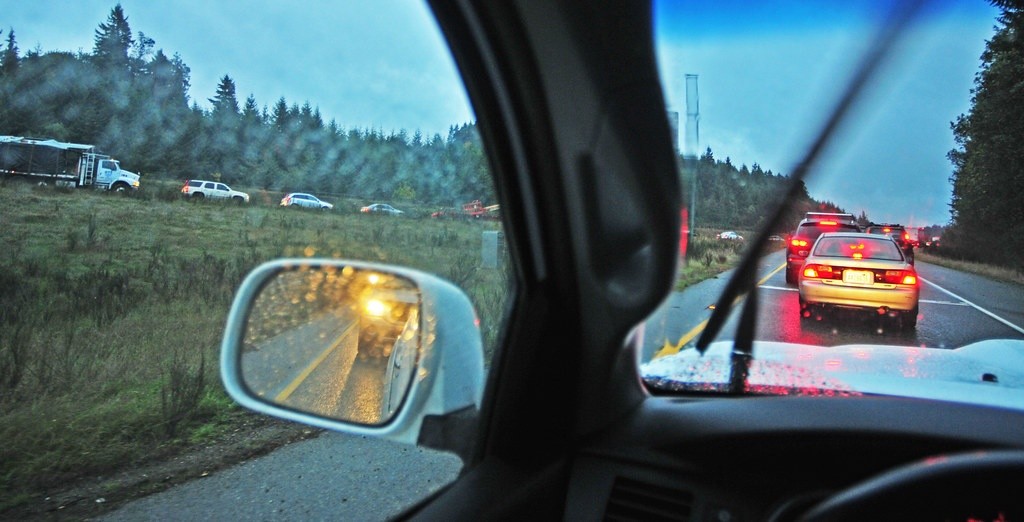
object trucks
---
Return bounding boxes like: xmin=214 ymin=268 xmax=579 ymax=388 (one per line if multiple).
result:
xmin=0 ymin=135 xmax=141 ymax=195
xmin=904 ymin=228 xmax=920 ymax=246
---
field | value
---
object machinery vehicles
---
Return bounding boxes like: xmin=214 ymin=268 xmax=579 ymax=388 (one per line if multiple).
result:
xmin=464 ymin=200 xmax=501 ymax=217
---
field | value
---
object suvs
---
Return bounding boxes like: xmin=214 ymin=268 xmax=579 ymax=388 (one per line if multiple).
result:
xmin=785 ymin=211 xmax=864 ymax=283
xmin=181 ymin=179 xmax=249 ymax=205
xmin=866 ymin=223 xmax=914 ymax=268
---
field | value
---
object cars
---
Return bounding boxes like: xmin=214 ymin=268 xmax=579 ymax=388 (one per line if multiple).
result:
xmin=361 ymin=203 xmax=404 ymax=216
xmin=281 ymin=193 xmax=334 ymax=211
xmin=797 ymin=231 xmax=920 ymax=325
xmin=717 ymin=231 xmax=744 ymax=240
xmin=768 ymin=234 xmax=786 ymax=242
